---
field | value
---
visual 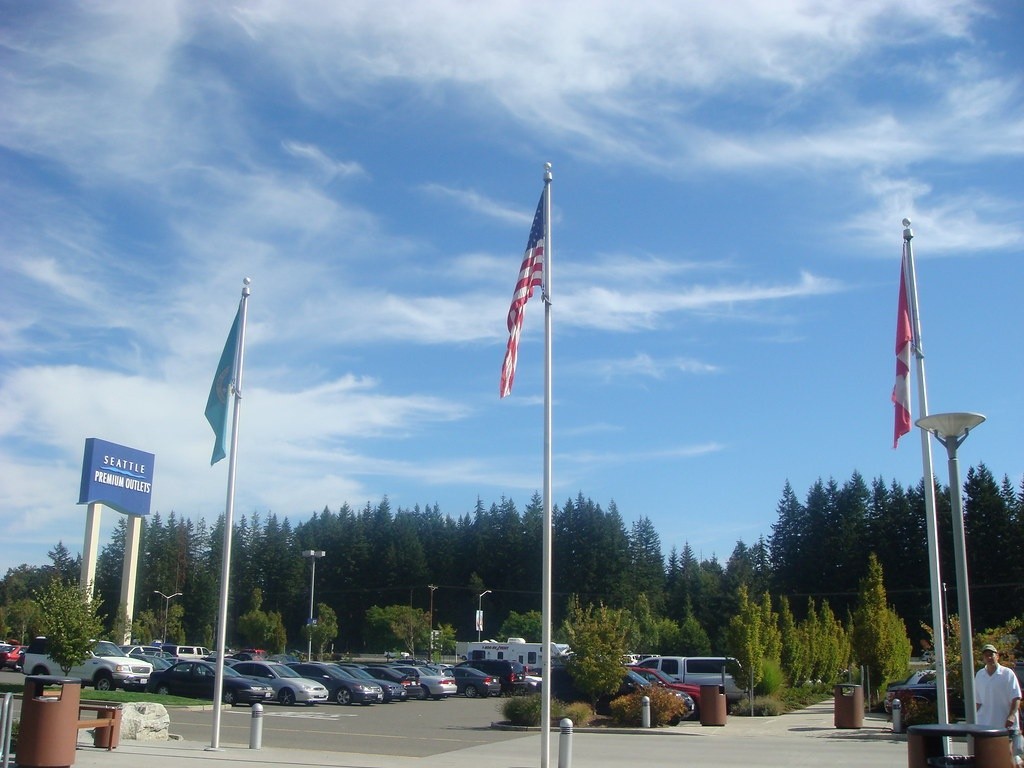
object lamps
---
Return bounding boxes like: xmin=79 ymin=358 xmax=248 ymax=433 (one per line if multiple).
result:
xmin=642 ymin=696 xmax=650 ymax=706
xmin=892 ymin=699 xmax=902 ymax=709
xmin=252 ymin=703 xmax=263 ymax=718
xmin=560 ymin=718 xmax=573 ymax=734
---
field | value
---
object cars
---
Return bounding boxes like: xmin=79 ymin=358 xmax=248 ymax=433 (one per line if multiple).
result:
xmin=150 ymin=661 xmax=275 ymax=708
xmin=883 ymin=661 xmax=1024 ymax=726
xmin=116 ymin=640 xmax=528 ymax=673
xmin=338 ymin=666 xmax=408 ymax=704
xmin=441 ymin=666 xmax=502 ymax=698
xmin=389 ymin=666 xmax=458 ymax=701
xmin=629 ymin=666 xmax=732 ymax=722
xmin=287 ymin=664 xmax=385 ymax=706
xmin=362 ymin=667 xmax=423 ymax=701
xmin=536 ymin=665 xmax=696 ymax=726
xmin=226 ymin=661 xmax=329 ymax=706
xmin=428 ymin=665 xmax=453 ymax=678
xmin=0 ymin=639 xmax=30 ymax=673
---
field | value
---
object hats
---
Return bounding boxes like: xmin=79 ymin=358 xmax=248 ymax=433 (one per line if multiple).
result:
xmin=982 ymin=645 xmax=997 ymax=654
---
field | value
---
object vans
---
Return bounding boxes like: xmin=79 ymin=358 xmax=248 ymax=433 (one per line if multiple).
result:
xmin=633 ymin=656 xmax=748 ymax=701
xmin=453 ymin=659 xmax=526 ymax=694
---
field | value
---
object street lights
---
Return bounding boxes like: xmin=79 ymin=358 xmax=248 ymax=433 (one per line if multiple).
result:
xmin=154 ymin=591 xmax=183 ymax=646
xmin=478 ymin=590 xmax=492 ymax=643
xmin=302 ymin=549 xmax=325 ymax=662
xmin=914 ymin=412 xmax=987 ymax=756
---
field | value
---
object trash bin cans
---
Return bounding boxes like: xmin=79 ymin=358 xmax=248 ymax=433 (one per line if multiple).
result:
xmin=700 ymin=684 xmax=726 ymax=726
xmin=93 ymin=709 xmax=122 ymax=748
xmin=907 ymin=724 xmax=1015 ymax=768
xmin=833 ymin=684 xmax=864 ymax=729
xmin=15 ymin=675 xmax=82 ymax=768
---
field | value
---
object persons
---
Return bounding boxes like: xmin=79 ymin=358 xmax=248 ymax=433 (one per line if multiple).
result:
xmin=975 ymin=644 xmax=1024 ymax=768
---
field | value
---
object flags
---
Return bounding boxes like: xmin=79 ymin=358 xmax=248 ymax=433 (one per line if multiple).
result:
xmin=205 ymin=304 xmax=240 ymax=466
xmin=500 ymin=188 xmax=546 ymax=397
xmin=892 ymin=253 xmax=913 ymax=449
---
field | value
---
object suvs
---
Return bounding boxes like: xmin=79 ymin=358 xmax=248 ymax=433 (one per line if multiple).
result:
xmin=22 ymin=636 xmax=153 ymax=691
